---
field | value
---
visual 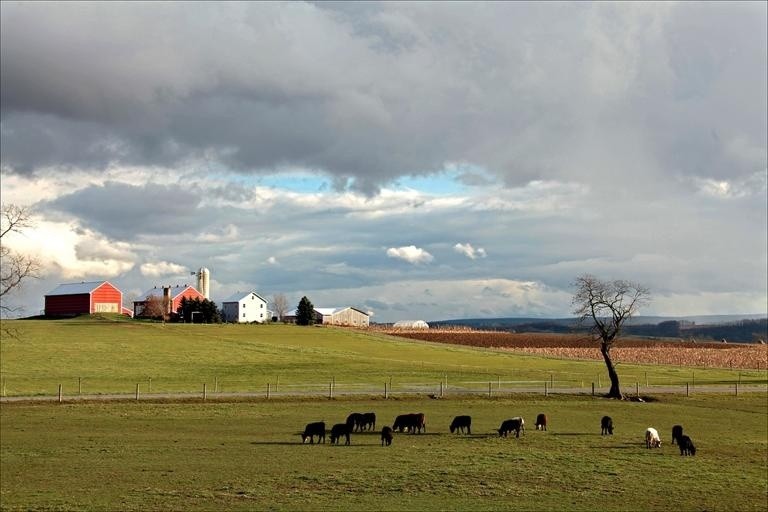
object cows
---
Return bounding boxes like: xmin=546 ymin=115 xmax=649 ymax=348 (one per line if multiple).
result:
xmin=345 ymin=412 xmax=376 ymax=433
xmin=644 ymin=428 xmax=662 ymax=449
xmin=329 ymin=424 xmax=351 ymax=446
xmin=534 ymin=413 xmax=546 ymax=431
xmin=449 ymin=415 xmax=471 ymax=435
xmin=672 ymin=425 xmax=683 ymax=445
xmin=496 ymin=416 xmax=525 ymax=438
xmin=301 ymin=422 xmax=325 ymax=444
xmin=381 ymin=426 xmax=393 ymax=447
xmin=676 ymin=435 xmax=696 ymax=456
xmin=601 ymin=416 xmax=614 ymax=435
xmin=392 ymin=413 xmax=426 ymax=435
